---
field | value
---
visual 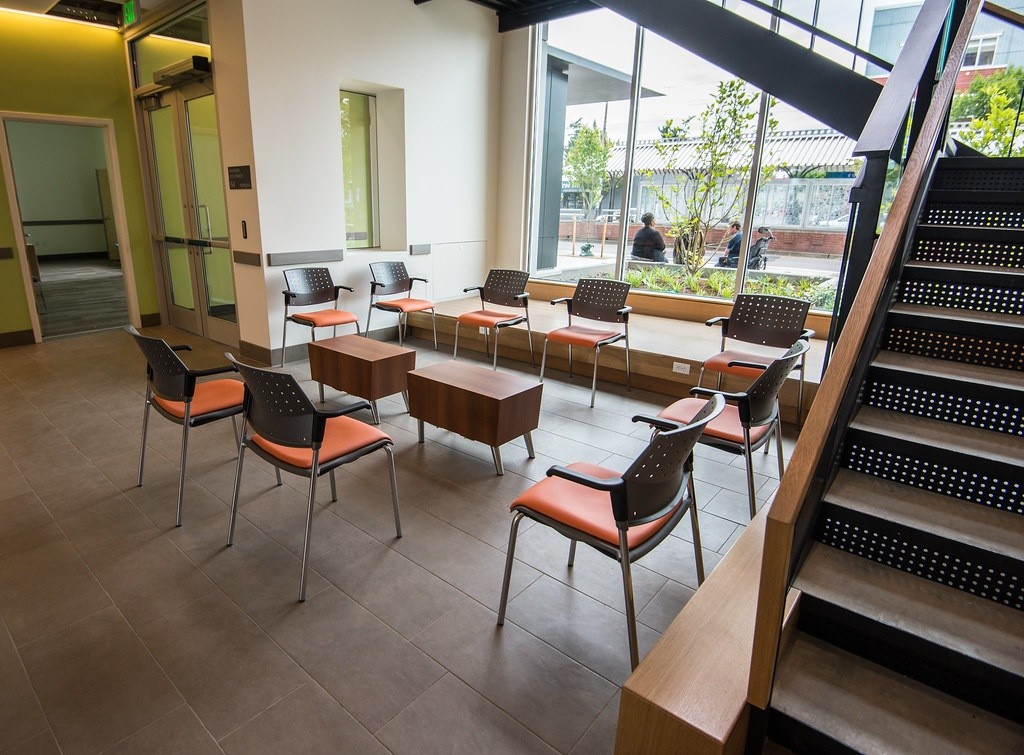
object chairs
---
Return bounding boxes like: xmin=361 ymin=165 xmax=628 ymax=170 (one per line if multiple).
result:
xmin=365 ymin=261 xmax=438 ymax=351
xmin=649 ymin=338 xmax=810 ymax=520
xmin=497 ymin=392 xmax=727 ymax=672
xmin=539 ymin=278 xmax=633 ymax=407
xmin=124 ymin=324 xmax=283 ymax=527
xmin=281 ymin=267 xmax=361 ymax=368
xmin=223 ymin=351 xmax=403 ymax=603
xmin=696 ymin=293 xmax=817 ymax=431
xmin=453 ymin=268 xmax=536 ymax=370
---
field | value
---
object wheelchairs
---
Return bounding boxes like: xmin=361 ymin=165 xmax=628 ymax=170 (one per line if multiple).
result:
xmin=717 ymin=235 xmax=776 ymax=271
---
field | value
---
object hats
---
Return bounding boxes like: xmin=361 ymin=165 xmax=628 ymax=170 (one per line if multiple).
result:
xmin=641 ymin=213 xmax=653 ymax=223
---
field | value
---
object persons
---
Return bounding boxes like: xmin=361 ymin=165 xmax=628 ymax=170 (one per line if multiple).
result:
xmin=714 ymin=221 xmax=743 ymax=267
xmin=631 ymin=212 xmax=669 ymax=262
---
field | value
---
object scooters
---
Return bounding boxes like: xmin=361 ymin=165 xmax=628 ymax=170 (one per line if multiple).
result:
xmin=579 ymin=243 xmax=595 ymax=257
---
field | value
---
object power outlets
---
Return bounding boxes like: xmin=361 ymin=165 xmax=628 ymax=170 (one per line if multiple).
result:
xmin=479 ymin=326 xmax=490 ymax=335
xmin=672 ymin=362 xmax=691 ymax=375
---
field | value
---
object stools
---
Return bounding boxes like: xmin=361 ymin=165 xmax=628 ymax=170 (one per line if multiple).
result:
xmin=32 ymin=275 xmax=48 ymax=314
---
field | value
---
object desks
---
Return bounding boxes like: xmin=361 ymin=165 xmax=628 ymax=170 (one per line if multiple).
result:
xmin=406 ymin=359 xmax=544 ymax=474
xmin=307 ymin=334 xmax=416 ymax=425
